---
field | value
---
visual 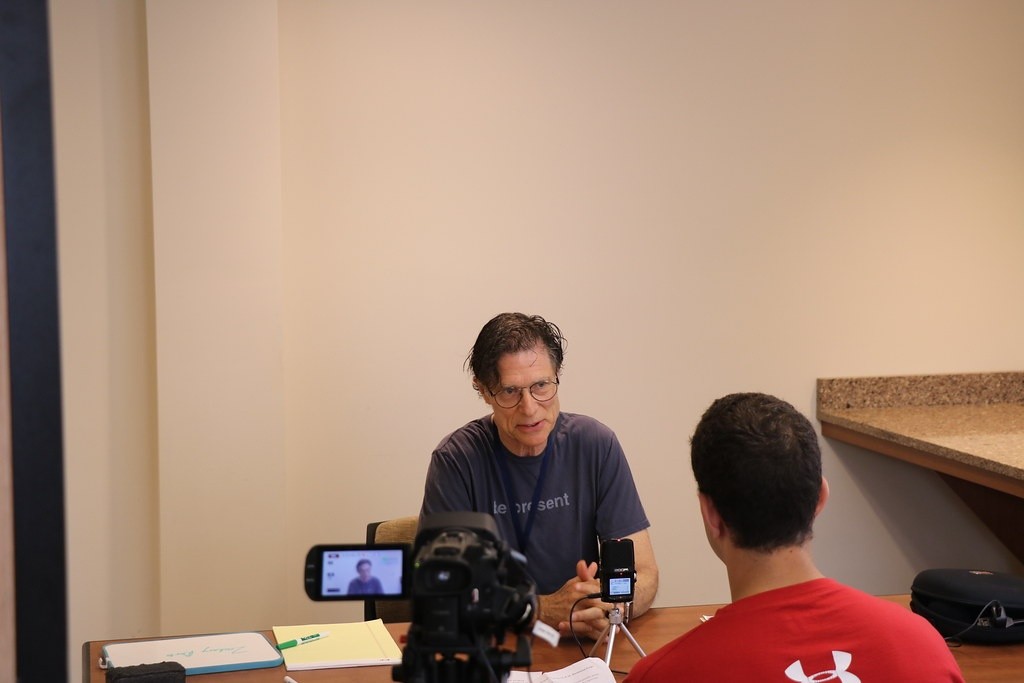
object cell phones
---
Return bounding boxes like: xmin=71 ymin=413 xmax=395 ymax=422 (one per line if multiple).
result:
xmin=598 ymin=538 xmax=635 ymax=601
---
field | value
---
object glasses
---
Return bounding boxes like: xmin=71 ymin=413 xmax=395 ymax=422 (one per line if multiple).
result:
xmin=485 ymin=374 xmax=559 ymax=410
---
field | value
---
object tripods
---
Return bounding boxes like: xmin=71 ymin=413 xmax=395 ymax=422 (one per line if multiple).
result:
xmin=584 ymin=601 xmax=649 ymax=672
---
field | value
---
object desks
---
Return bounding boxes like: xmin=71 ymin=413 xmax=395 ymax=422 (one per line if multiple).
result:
xmin=85 ymin=592 xmax=1024 ymax=683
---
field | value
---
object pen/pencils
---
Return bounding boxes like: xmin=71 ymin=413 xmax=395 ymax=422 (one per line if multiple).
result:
xmin=283 ymin=675 xmax=297 ymax=683
xmin=276 ymin=630 xmax=329 ymax=650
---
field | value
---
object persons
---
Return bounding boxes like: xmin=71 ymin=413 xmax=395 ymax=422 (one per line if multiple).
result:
xmin=622 ymin=392 xmax=965 ymax=683
xmin=412 ymin=310 xmax=660 ymax=644
xmin=346 ymin=558 xmax=384 ymax=595
